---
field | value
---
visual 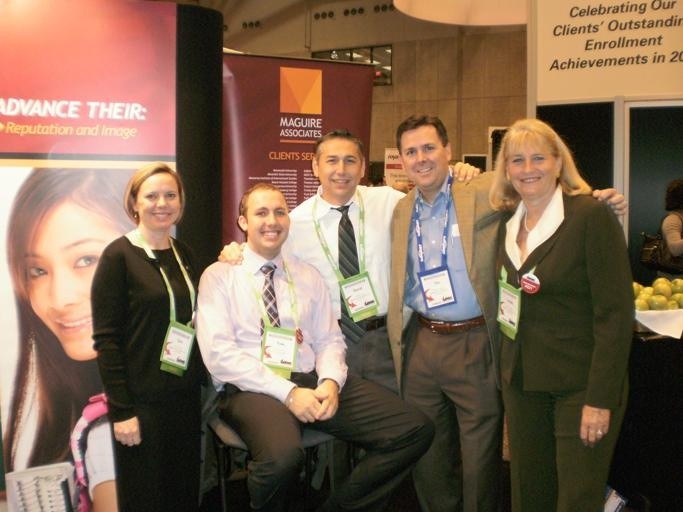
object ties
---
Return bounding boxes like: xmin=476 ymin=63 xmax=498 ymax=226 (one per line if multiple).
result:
xmin=331 ymin=202 xmax=359 ymax=278
xmin=260 ymin=264 xmax=281 ymax=328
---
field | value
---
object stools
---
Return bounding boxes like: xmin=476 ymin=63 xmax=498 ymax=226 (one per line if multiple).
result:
xmin=208 ymin=393 xmax=339 ymax=507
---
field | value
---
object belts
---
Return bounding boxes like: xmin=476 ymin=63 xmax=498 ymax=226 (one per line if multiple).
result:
xmin=417 ymin=314 xmax=485 ymax=335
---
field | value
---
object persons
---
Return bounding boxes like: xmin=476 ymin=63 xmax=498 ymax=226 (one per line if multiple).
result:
xmin=659 ymin=178 xmax=683 ymax=279
xmin=488 ymin=117 xmax=636 ymax=512
xmin=8 ymin=169 xmax=137 ymax=512
xmin=388 ymin=113 xmax=628 ymax=512
xmin=195 ymin=181 xmax=435 ymax=512
xmin=216 ymin=128 xmax=480 ymax=394
xmin=89 ymin=162 xmax=204 ymax=512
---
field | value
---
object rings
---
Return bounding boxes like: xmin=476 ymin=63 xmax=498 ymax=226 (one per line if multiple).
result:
xmin=596 ymin=429 xmax=603 ymax=437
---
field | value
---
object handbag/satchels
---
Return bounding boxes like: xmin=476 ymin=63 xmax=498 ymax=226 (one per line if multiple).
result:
xmin=640 ymin=212 xmax=683 ymax=271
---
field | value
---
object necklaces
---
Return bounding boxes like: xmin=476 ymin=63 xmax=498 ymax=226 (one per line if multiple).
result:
xmin=520 ymin=210 xmax=534 ymax=233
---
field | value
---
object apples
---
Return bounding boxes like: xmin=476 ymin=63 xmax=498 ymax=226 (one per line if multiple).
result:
xmin=648 ymin=294 xmax=667 ymax=309
xmin=652 ymin=278 xmax=671 ymax=286
xmin=670 ymin=278 xmax=683 ymax=293
xmin=670 ymin=292 xmax=683 ymax=307
xmin=637 ymin=294 xmax=651 ymax=301
xmin=632 ymin=281 xmax=640 ymax=298
xmin=641 ymin=287 xmax=654 ymax=295
xmin=668 ymin=300 xmax=679 ymax=310
xmin=635 ymin=299 xmax=650 ymax=310
xmin=654 ymin=284 xmax=672 ymax=297
